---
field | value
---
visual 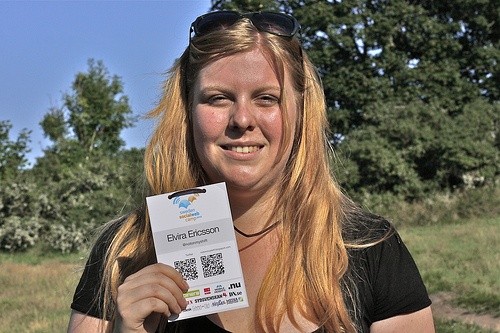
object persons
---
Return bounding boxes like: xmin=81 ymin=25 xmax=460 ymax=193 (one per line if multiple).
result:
xmin=68 ymin=8 xmax=435 ymax=332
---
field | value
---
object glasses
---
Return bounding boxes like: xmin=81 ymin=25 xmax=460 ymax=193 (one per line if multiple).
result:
xmin=188 ymin=11 xmax=303 ymax=55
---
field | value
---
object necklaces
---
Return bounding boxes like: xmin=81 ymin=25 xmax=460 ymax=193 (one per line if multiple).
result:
xmin=232 ymin=220 xmax=279 ymax=239
